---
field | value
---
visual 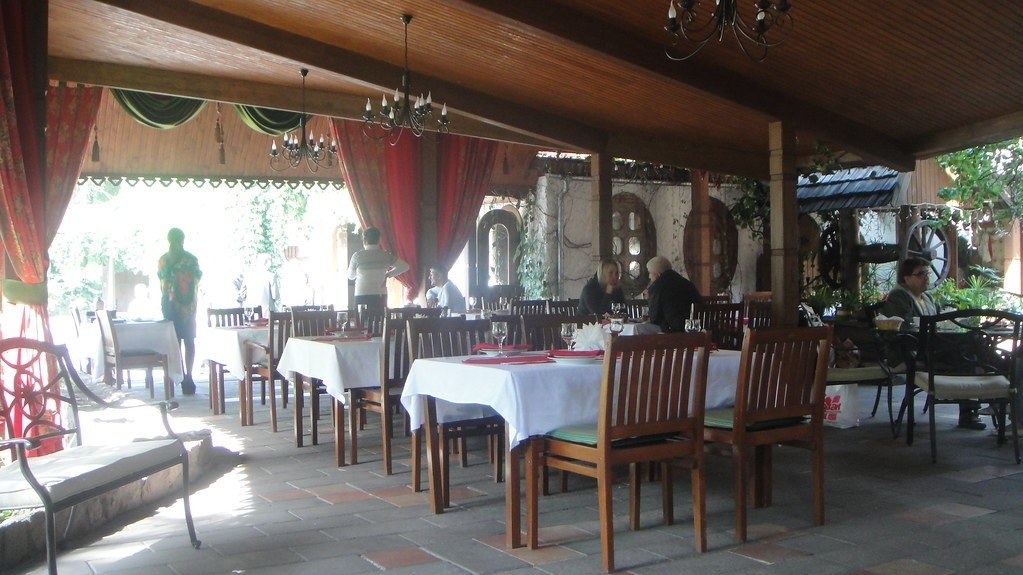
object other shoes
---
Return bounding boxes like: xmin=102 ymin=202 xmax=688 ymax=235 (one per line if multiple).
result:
xmin=958 ymin=412 xmax=987 ymax=430
xmin=181 ymin=376 xmax=196 ymax=393
xmin=979 ymin=405 xmax=996 ymax=414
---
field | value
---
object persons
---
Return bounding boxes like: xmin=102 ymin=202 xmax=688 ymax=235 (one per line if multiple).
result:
xmin=576 ymin=259 xmax=626 ymax=317
xmin=647 ymin=256 xmax=706 ymax=332
xmin=158 ymin=227 xmax=202 ymax=393
xmin=883 ymin=257 xmax=1023 ymax=436
xmin=228 ymin=253 xmax=320 ymax=316
xmin=347 ymin=227 xmax=409 ymax=320
xmin=426 ymin=265 xmax=467 ymax=312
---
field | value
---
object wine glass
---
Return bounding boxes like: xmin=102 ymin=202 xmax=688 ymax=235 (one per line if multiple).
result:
xmin=469 ymin=296 xmax=477 ymax=312
xmin=491 ymin=322 xmax=508 ymax=358
xmin=337 ymin=312 xmax=348 ymax=340
xmin=561 ymin=323 xmax=577 ymax=352
xmin=499 ymin=296 xmax=507 ymax=313
xmin=244 ymin=304 xmax=253 ymax=328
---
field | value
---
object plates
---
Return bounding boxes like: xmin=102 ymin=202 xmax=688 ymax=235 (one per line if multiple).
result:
xmin=247 ymin=322 xmax=268 ymax=327
xmin=470 ymin=346 xmax=598 ymax=364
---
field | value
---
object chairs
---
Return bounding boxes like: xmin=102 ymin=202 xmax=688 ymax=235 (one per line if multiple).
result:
xmin=0 ymin=290 xmax=1023 ymax=575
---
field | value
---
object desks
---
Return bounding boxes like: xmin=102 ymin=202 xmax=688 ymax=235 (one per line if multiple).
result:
xmin=568 ymin=320 xmax=661 ymax=348
xmin=862 ymin=326 xmax=1023 ymax=435
xmin=199 ymin=320 xmax=355 ymax=426
xmin=83 ymin=318 xmax=183 ymax=401
xmin=276 ymin=331 xmax=493 ymax=462
xmin=401 ymin=346 xmax=781 ymax=550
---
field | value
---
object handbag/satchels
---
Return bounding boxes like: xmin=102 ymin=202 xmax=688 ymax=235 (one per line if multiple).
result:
xmin=806 ymin=383 xmax=860 ymax=429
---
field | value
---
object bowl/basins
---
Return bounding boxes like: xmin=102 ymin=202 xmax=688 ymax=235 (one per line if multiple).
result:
xmin=874 ymin=318 xmax=904 ymax=330
xmin=329 ymin=330 xmax=362 ymax=337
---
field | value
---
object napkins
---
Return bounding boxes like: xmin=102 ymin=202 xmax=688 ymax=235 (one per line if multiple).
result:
xmin=324 ymin=327 xmax=367 ymax=332
xmin=470 ymin=342 xmax=534 ymax=351
xmin=464 ymin=355 xmax=551 ymax=364
xmin=316 ymin=336 xmax=369 ymax=342
xmin=255 ymin=319 xmax=268 ymax=327
xmin=546 ymin=349 xmax=604 ymax=357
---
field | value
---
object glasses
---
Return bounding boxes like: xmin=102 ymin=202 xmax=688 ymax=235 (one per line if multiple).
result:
xmin=912 ymin=271 xmax=932 ymax=278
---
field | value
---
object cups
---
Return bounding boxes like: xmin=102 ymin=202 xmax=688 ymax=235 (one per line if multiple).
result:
xmin=611 ymin=302 xmax=620 ymax=314
xmin=685 ymin=319 xmax=701 ymax=332
xmin=912 ymin=316 xmax=921 ymax=327
xmin=641 ymin=307 xmax=649 ymax=321
xmin=611 ymin=319 xmax=623 ymax=334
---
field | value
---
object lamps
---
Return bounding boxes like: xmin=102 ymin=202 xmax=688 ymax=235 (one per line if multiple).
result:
xmin=360 ymin=14 xmax=450 ymax=146
xmin=269 ymin=68 xmax=339 ymax=173
xmin=663 ymin=0 xmax=794 ymax=64
